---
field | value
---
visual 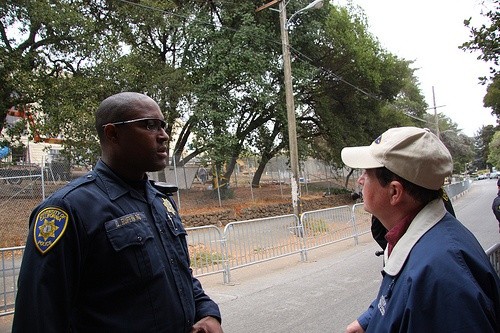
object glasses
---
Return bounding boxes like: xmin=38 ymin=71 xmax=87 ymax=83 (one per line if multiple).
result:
xmin=101 ymin=118 xmax=166 ymax=132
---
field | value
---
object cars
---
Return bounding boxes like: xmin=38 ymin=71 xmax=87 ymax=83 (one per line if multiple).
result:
xmin=478 ymin=173 xmax=499 ymax=180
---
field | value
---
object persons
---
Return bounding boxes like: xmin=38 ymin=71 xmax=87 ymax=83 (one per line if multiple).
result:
xmin=198 ymin=165 xmax=208 ymax=186
xmin=371 ymin=186 xmax=457 ymax=280
xmin=339 ymin=126 xmax=500 ymax=333
xmin=491 ymin=177 xmax=500 ymax=233
xmin=16 ymin=157 xmax=23 ymax=163
xmin=12 ymin=93 xmax=223 ymax=333
xmin=211 ymin=173 xmax=229 ymax=199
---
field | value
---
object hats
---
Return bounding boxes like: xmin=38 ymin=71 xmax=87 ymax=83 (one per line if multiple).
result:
xmin=340 ymin=126 xmax=454 ymax=189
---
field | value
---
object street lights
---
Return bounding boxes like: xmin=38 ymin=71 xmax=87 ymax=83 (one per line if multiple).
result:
xmin=288 ymin=0 xmax=324 ymax=24
